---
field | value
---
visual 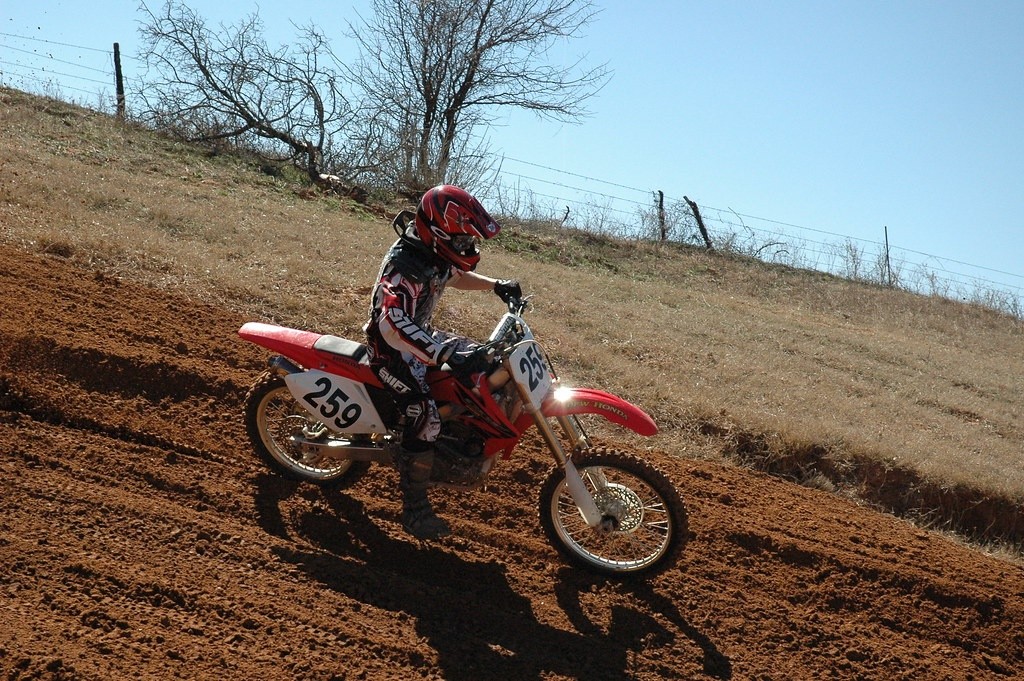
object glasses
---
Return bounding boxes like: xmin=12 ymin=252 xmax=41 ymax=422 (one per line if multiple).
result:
xmin=451 ymin=234 xmax=475 ymax=250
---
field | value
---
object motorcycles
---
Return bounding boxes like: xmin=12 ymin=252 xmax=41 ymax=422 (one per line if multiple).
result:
xmin=238 ymin=293 xmax=691 ymax=582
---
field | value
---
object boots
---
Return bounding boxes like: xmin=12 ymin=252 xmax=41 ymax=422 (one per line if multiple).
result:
xmin=396 ymin=444 xmax=451 ymax=541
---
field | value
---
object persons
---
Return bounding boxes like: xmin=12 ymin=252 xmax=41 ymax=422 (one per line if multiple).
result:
xmin=364 ymin=185 xmax=522 ymax=538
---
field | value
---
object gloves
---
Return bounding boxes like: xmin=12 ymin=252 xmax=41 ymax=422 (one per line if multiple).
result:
xmin=463 ymin=344 xmax=498 ymax=374
xmin=494 ymin=279 xmax=522 ymax=304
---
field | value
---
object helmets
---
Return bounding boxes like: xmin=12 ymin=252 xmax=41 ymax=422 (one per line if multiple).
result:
xmin=416 ymin=185 xmax=500 ymax=272
